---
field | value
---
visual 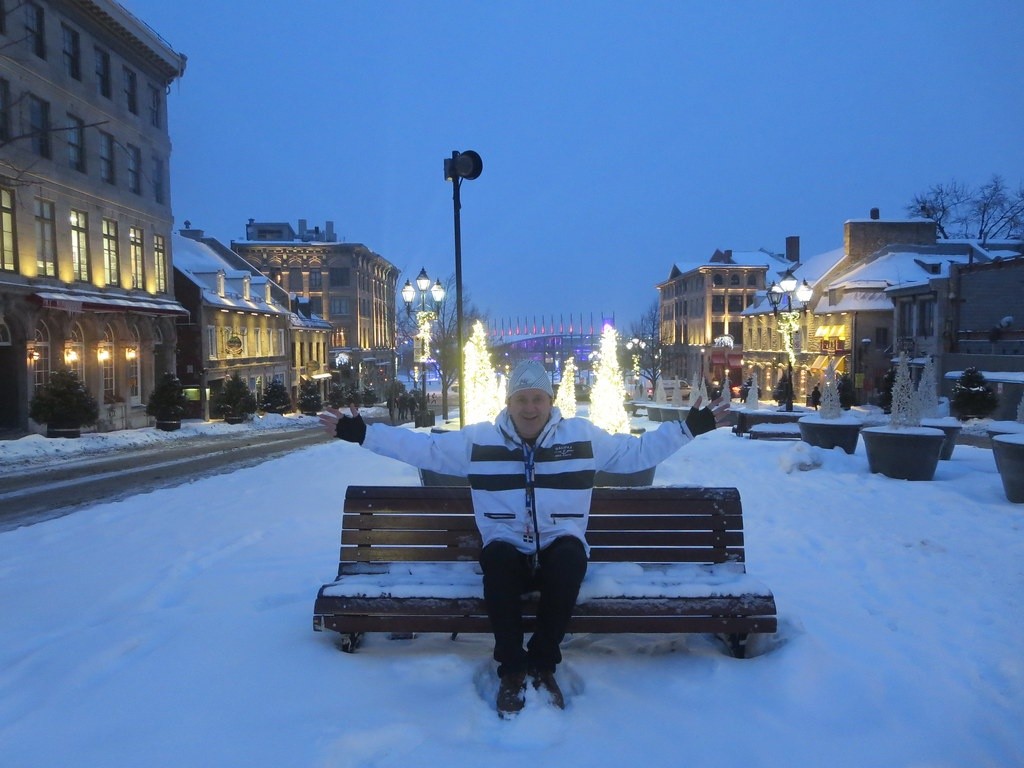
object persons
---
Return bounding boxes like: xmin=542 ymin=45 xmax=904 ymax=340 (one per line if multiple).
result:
xmin=386 ymin=390 xmax=438 ymax=419
xmin=319 ymin=360 xmax=732 ymax=717
xmin=812 ymin=387 xmax=821 ymax=411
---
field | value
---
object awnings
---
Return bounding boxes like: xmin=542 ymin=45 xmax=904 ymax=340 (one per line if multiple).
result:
xmin=32 ymin=292 xmax=190 ymax=317
xmin=811 ymin=356 xmax=845 ymax=372
xmin=813 ymin=324 xmax=845 ymax=338
xmin=712 ymin=355 xmax=743 ymax=368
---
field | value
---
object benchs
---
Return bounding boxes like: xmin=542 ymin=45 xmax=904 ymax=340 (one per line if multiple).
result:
xmin=312 ymin=485 xmax=778 ymax=659
xmin=732 ymin=412 xmax=805 ymax=441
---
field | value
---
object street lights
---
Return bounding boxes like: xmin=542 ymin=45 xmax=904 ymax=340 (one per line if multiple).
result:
xmin=765 ymin=267 xmax=815 ymax=412
xmin=625 ymin=337 xmax=647 ymax=393
xmin=400 ymin=264 xmax=446 ymax=429
xmin=442 ymin=149 xmax=484 ymax=431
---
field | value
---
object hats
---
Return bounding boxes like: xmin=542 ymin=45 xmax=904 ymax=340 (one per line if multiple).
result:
xmin=507 ymin=360 xmax=554 ymax=401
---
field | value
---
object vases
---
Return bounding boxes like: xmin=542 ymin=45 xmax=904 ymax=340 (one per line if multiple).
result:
xmin=992 ymin=436 xmax=1024 ymax=502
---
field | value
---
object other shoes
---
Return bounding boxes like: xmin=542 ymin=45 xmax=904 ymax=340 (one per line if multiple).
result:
xmin=496 ymin=670 xmax=526 ymax=719
xmin=527 ymin=665 xmax=565 ymax=710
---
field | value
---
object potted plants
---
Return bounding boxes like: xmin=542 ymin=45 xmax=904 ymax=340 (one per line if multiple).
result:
xmin=985 ymin=394 xmax=1024 ymax=473
xmin=588 ymin=323 xmax=656 ymax=485
xmin=215 ymin=370 xmax=256 ymax=424
xmin=259 ymin=378 xmax=292 ymax=416
xmin=144 ymin=369 xmax=191 ymax=431
xmin=361 ymin=386 xmax=378 ymax=408
xmin=327 ymin=383 xmax=345 ymax=410
xmin=859 ymin=351 xmax=963 ymax=480
xmin=296 ymin=379 xmax=323 ymax=416
xmin=26 ymin=366 xmax=99 ymax=438
xmin=797 ymin=361 xmax=863 ymax=454
xmin=346 ymin=390 xmax=361 ymax=411
xmin=419 ymin=320 xmax=506 ymax=485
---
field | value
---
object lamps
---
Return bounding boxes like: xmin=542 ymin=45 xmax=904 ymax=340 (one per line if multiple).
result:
xmin=29 ymin=351 xmax=39 ymax=361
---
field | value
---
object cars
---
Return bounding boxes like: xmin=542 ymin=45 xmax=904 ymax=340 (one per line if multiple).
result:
xmin=648 ymin=378 xmax=692 ymax=401
